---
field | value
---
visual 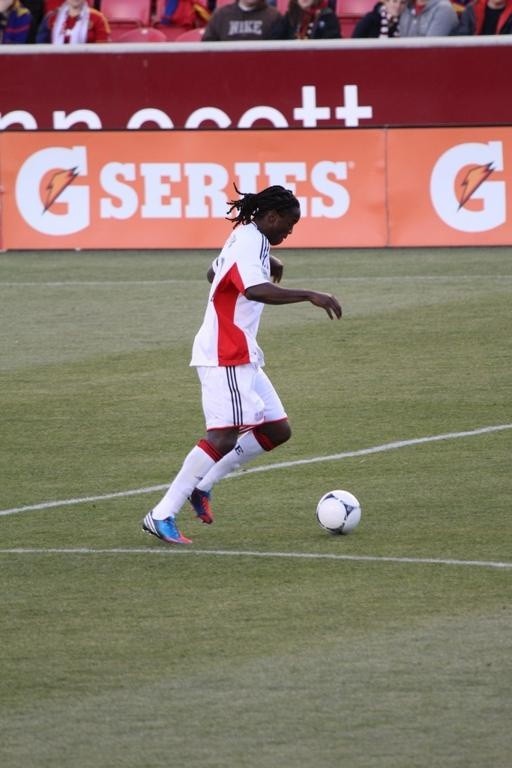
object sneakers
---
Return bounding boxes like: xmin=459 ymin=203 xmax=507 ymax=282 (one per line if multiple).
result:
xmin=142 ymin=511 xmax=192 ymax=545
xmin=188 ymin=488 xmax=213 ymax=523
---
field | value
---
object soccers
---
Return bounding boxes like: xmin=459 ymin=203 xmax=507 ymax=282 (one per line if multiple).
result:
xmin=317 ymin=490 xmax=362 ymax=534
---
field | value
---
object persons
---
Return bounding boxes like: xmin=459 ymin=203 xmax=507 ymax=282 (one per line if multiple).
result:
xmin=201 ymin=0 xmax=282 ymax=40
xmin=399 ymin=0 xmax=460 ymax=38
xmin=459 ymin=0 xmax=512 ymax=36
xmin=143 ymin=187 xmax=342 ymax=546
xmin=272 ymin=0 xmax=341 ymax=40
xmin=351 ymin=0 xmax=408 ymax=38
xmin=0 ymin=1 xmax=32 ymax=45
xmin=36 ymin=0 xmax=111 ymax=44
